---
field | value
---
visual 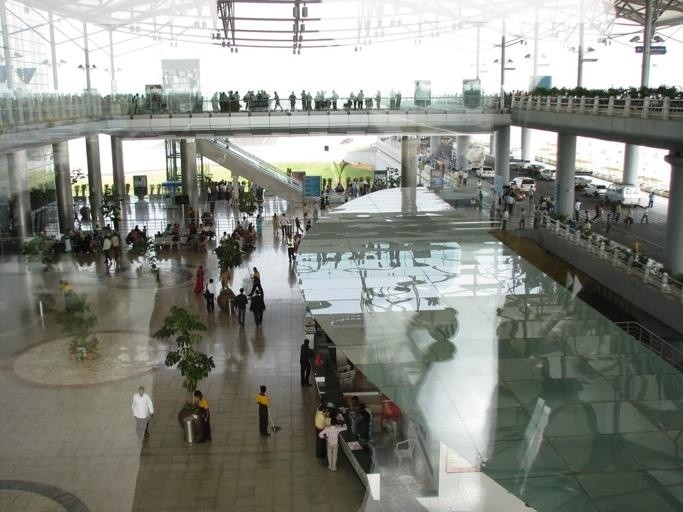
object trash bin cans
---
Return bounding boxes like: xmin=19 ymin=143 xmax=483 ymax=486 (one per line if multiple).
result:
xmin=178 ymin=409 xmax=203 ymax=443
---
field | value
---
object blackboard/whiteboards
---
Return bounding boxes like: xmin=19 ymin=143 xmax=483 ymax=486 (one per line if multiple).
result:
xmin=302 ymin=176 xmax=322 ymax=196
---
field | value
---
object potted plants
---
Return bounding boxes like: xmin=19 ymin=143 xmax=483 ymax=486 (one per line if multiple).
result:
xmin=153 ymin=302 xmax=216 ymax=444
xmin=54 ymin=288 xmax=101 ymax=361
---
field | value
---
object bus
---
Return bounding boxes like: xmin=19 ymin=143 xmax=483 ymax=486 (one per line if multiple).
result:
xmin=451 ymin=146 xmax=485 ymax=168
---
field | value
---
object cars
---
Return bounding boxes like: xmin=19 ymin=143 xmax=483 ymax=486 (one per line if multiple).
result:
xmin=508 ymin=157 xmax=640 ymax=208
xmin=475 ymin=166 xmax=495 ymax=178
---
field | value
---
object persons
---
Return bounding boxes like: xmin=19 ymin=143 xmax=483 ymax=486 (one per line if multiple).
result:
xmin=132 ymin=387 xmax=154 ymax=440
xmin=1 ymin=85 xmax=683 ymax=328
xmin=194 ymin=390 xmax=211 ymax=434
xmin=300 ymin=339 xmax=370 ymax=471
xmin=256 ymin=386 xmax=272 ymax=435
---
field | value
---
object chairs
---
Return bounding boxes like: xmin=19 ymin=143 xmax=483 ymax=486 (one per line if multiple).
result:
xmin=394 ymin=438 xmax=419 ymax=466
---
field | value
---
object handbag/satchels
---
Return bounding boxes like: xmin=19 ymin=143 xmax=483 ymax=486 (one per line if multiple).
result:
xmin=204 ymin=289 xmax=211 ymax=299
